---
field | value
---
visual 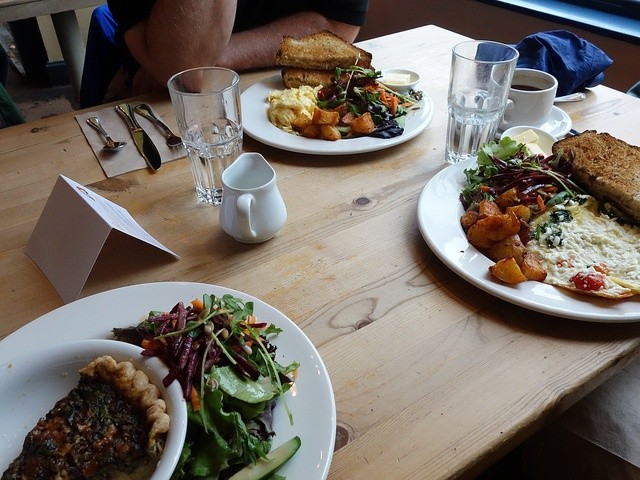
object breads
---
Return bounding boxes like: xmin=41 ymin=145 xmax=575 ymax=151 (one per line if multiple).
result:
xmin=274 ymin=30 xmax=374 ymax=92
xmin=552 ymin=130 xmax=640 ymax=219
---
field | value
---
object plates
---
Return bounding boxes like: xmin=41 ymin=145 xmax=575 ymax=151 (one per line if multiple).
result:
xmin=239 ymin=71 xmax=434 ymax=155
xmin=0 ymin=280 xmax=337 ymax=476
xmin=500 ymin=125 xmax=557 ymax=160
xmin=377 ymin=69 xmax=422 ymax=93
xmin=1 ymin=338 xmax=189 ymax=476
xmin=418 ymin=153 xmax=638 ymax=324
xmin=488 ymin=106 xmax=574 ymax=137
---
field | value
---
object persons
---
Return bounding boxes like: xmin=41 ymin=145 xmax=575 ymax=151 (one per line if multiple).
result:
xmin=104 ymin=0 xmax=369 ymax=101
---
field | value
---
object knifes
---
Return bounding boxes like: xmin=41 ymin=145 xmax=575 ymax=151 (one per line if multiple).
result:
xmin=114 ymin=102 xmax=161 ymax=173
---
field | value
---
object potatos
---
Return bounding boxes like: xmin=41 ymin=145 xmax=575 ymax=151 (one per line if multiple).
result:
xmin=290 ymin=107 xmax=375 ymax=141
xmin=462 ymin=189 xmax=549 ymax=284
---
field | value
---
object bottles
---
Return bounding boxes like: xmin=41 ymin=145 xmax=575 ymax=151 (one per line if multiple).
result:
xmin=219 ymin=152 xmax=287 ymax=245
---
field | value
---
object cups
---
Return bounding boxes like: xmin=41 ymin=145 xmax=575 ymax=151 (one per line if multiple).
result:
xmin=167 ymin=66 xmax=244 ymax=206
xmin=444 ymin=41 xmax=519 ymax=165
xmin=499 ymin=67 xmax=558 ymax=130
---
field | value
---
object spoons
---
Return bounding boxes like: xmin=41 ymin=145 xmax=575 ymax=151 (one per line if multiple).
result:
xmin=85 ymin=116 xmax=127 ymax=152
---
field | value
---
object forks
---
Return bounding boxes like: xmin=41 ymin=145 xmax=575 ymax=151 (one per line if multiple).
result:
xmin=134 ymin=102 xmax=184 ymax=147
